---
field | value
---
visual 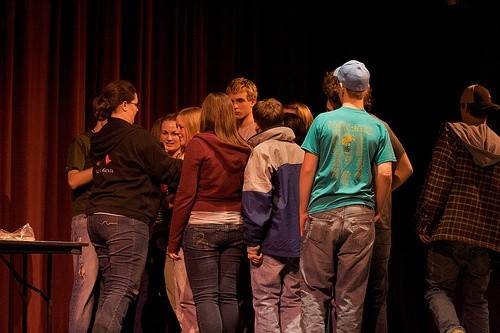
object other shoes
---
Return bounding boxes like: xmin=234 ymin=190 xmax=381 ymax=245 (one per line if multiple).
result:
xmin=451 ymin=329 xmax=465 ymax=333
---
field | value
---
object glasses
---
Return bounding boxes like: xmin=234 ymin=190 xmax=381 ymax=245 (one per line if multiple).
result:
xmin=120 ymin=101 xmax=141 ymax=109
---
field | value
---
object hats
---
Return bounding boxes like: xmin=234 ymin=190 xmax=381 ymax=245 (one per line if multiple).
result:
xmin=333 ymin=60 xmax=370 ymax=91
xmin=460 ymin=84 xmax=498 ymax=107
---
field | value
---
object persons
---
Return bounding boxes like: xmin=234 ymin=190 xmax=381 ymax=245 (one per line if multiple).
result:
xmin=141 ymin=106 xmax=202 ymax=333
xmin=167 ymin=92 xmax=253 ymax=333
xmin=85 ymin=79 xmax=184 ymax=333
xmin=416 ymin=85 xmax=500 ymax=333
xmin=299 ymin=60 xmax=413 ymax=333
xmin=65 ymin=119 xmax=102 ymax=333
xmin=226 ymin=78 xmax=314 ymax=333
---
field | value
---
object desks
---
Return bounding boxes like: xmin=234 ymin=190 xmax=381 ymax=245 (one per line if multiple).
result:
xmin=0 ymin=240 xmax=89 ymax=333
